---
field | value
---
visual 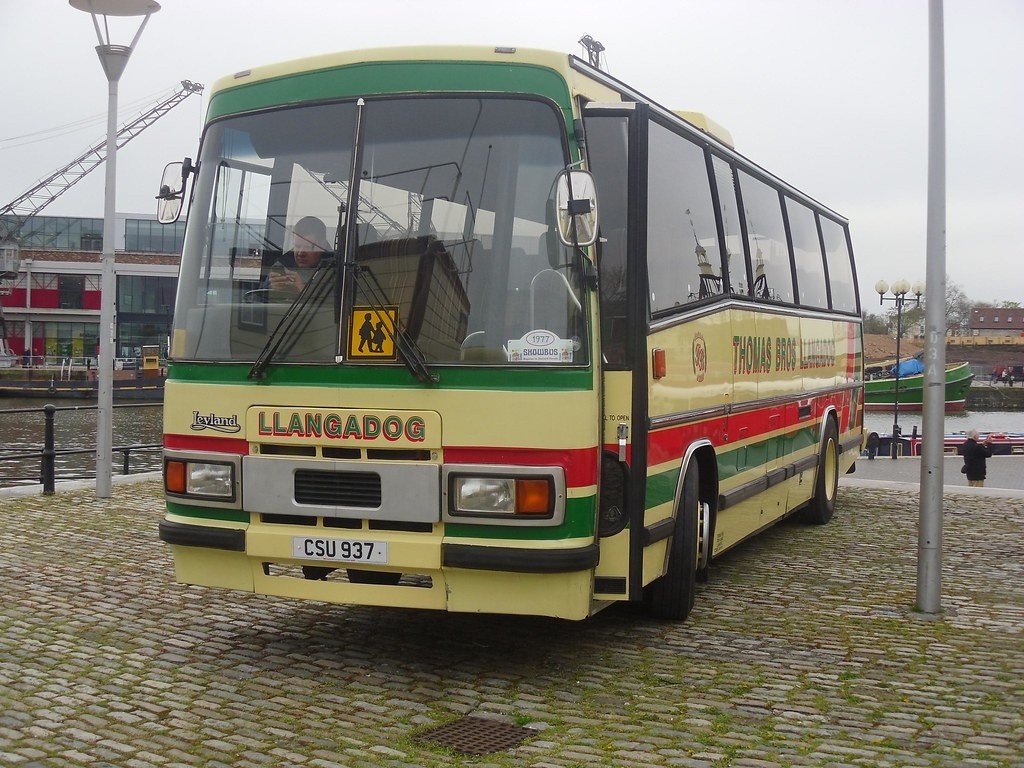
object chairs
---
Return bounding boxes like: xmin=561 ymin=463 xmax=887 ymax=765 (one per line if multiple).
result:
xmin=350 ymin=222 xmax=559 ymax=331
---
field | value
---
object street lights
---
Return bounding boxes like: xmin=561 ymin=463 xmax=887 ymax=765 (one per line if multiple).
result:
xmin=875 ymin=277 xmax=925 ymax=459
xmin=71 ymin=0 xmax=163 ymax=503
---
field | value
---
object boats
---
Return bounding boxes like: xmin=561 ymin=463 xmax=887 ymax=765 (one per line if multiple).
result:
xmin=864 ymin=361 xmax=974 ymax=412
xmin=862 ymin=431 xmax=1024 ymax=455
xmin=0 ymin=345 xmax=165 ymax=400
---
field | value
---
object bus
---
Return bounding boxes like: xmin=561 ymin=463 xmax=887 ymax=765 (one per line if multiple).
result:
xmin=156 ymin=46 xmax=862 ymax=626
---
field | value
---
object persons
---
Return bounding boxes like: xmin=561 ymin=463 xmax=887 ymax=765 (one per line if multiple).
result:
xmin=991 ymin=367 xmax=1013 ymax=386
xmin=253 ymin=217 xmax=336 ymax=305
xmin=23 ymin=346 xmax=39 ymax=368
xmin=961 ymin=429 xmax=993 ymax=487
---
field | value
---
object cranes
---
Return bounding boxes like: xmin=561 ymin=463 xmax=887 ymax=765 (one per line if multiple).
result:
xmin=0 ymin=79 xmax=206 ymax=369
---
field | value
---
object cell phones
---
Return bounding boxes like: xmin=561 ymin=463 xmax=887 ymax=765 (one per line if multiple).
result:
xmin=272 ymin=265 xmax=286 ymax=276
